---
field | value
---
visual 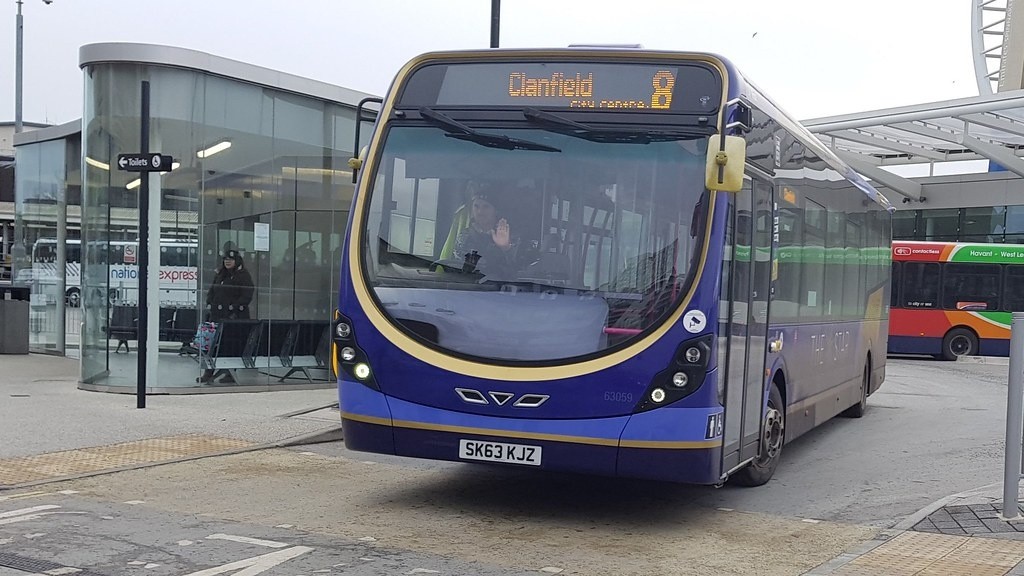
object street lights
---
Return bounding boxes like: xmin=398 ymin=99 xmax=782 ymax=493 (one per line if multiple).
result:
xmin=9 ymin=0 xmax=53 ymax=282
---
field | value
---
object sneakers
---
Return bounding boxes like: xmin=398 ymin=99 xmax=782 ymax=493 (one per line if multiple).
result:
xmin=195 ymin=371 xmax=214 ymax=382
xmin=218 ymin=373 xmax=235 ymax=383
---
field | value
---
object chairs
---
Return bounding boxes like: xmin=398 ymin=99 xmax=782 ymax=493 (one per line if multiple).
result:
xmin=99 ymin=304 xmax=336 ymax=386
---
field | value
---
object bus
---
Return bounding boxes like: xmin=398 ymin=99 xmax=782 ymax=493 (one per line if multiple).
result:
xmin=31 ymin=238 xmax=200 ymax=311
xmin=710 ymin=242 xmax=891 ymax=365
xmin=885 ymin=240 xmax=1024 ymax=365
xmin=335 ymin=42 xmax=896 ymax=491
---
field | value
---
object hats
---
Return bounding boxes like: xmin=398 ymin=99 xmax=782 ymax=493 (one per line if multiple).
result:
xmin=470 ymin=182 xmax=500 ymax=208
xmin=222 ymin=250 xmax=241 ymax=260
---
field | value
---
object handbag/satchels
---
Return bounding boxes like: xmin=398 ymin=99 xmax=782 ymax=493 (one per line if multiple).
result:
xmin=192 ymin=308 xmax=220 ymax=352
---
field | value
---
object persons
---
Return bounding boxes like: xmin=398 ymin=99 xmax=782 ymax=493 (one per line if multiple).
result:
xmin=449 ymin=189 xmax=524 ymax=285
xmin=193 ymin=248 xmax=255 ymax=381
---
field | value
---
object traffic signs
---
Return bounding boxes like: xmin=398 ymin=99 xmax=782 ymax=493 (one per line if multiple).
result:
xmin=117 ymin=152 xmax=162 ymax=170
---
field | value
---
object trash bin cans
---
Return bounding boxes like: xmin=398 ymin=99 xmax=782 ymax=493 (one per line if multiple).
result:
xmin=0 ymin=281 xmax=32 ymax=356
